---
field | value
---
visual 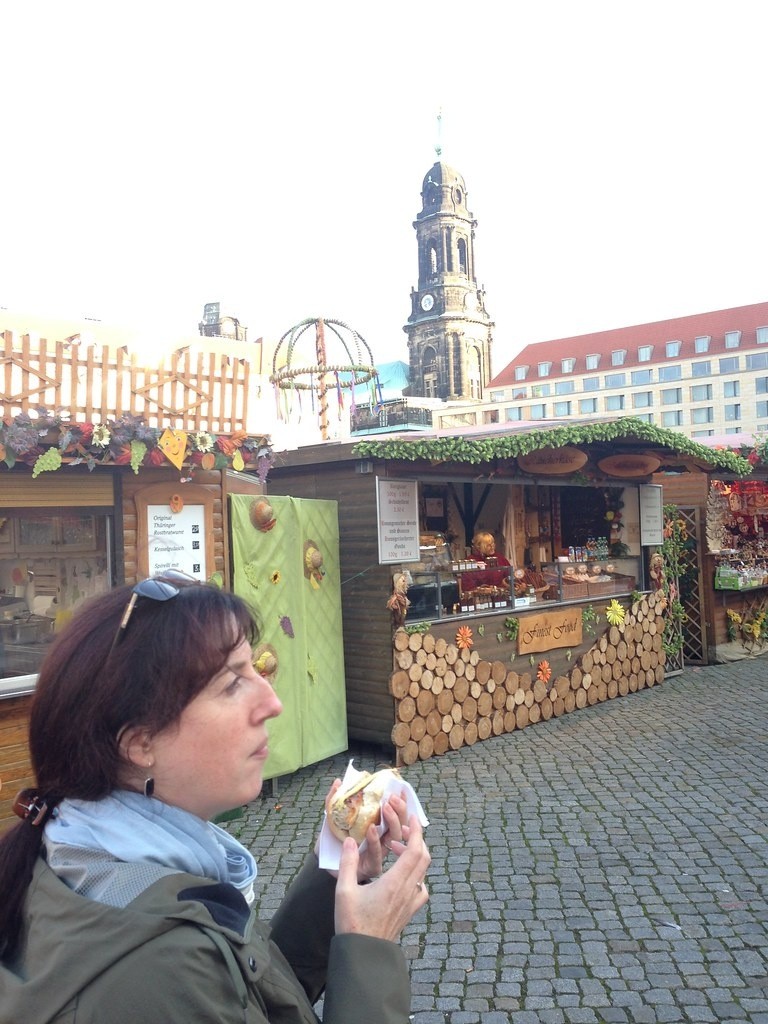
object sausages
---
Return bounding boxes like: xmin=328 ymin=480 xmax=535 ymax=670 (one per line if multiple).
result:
xmin=512 ymin=568 xmax=543 ymax=589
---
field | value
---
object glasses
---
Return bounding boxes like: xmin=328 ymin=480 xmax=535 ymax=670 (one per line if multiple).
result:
xmin=104 ymin=569 xmax=198 ymax=663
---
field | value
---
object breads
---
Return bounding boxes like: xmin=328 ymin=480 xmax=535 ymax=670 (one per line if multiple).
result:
xmin=392 ymin=535 xmax=453 ymax=584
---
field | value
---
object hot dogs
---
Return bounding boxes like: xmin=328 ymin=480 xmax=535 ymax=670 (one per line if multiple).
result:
xmin=325 ymin=768 xmax=402 ymax=845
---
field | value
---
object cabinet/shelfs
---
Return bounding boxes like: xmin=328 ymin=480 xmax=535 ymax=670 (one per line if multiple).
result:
xmin=0 ymin=516 xmax=104 ymax=558
xmin=510 ymin=485 xmax=560 ymax=576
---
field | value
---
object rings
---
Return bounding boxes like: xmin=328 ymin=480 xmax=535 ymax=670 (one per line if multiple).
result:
xmin=383 ymin=836 xmax=393 ymax=851
xmin=416 ymin=883 xmax=422 ymax=892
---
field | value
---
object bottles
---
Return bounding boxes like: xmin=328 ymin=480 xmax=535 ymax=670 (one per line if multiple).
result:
xmin=586 ymin=537 xmax=609 ymax=561
xmin=461 ymin=589 xmax=507 ymax=611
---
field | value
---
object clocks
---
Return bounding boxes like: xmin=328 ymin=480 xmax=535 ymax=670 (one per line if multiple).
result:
xmin=420 ymin=293 xmax=434 ymax=313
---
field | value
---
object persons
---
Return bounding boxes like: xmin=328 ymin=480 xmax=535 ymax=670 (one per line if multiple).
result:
xmin=0 ymin=580 xmax=431 ymax=1024
xmin=649 ymin=553 xmax=667 ymax=589
xmin=733 ymin=516 xmax=758 ymax=550
xmin=386 ymin=573 xmax=411 ymax=627
xmin=461 ymin=531 xmax=527 ymax=603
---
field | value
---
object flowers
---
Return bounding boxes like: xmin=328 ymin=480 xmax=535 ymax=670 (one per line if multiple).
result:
xmin=195 ymin=430 xmax=214 ymax=454
xmin=726 ymin=606 xmax=768 ymax=640
xmin=456 ymin=626 xmax=475 ymax=650
xmin=605 ymin=599 xmax=625 ymax=626
xmin=91 ymin=422 xmax=111 ymax=449
xmin=535 ymin=658 xmax=553 ymax=682
